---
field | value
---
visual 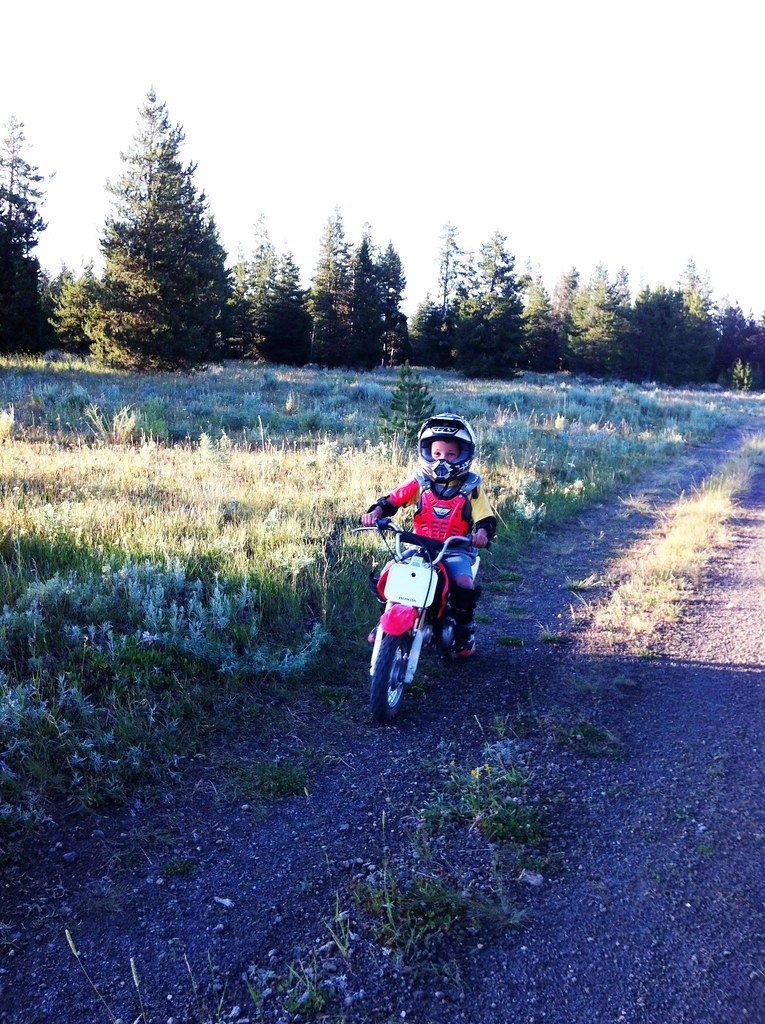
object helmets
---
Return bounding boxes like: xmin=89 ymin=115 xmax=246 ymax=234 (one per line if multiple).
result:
xmin=418 ymin=413 xmax=475 ymax=484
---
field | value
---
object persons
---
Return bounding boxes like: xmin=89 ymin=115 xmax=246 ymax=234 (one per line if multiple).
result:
xmin=361 ymin=412 xmax=497 ymax=657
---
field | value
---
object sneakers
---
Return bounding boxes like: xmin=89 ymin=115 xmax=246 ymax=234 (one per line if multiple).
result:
xmin=367 ymin=622 xmax=380 ymax=646
xmin=452 ymin=619 xmax=476 ymax=658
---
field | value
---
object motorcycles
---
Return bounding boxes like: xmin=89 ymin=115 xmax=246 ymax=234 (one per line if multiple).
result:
xmin=351 ymin=513 xmax=493 ymax=724
